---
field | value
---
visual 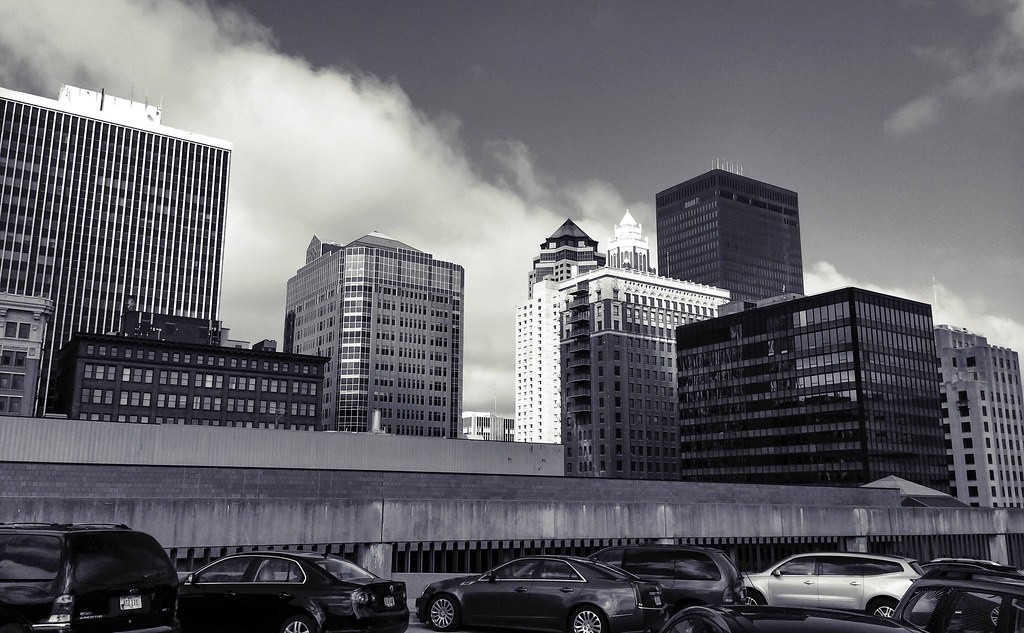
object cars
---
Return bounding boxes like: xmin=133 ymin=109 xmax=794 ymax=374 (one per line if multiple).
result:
xmin=890 ymin=569 xmax=1024 ymax=633
xmin=416 ymin=556 xmax=669 ymax=633
xmin=175 ymin=549 xmax=410 ymax=633
xmin=658 ymin=604 xmax=929 ymax=633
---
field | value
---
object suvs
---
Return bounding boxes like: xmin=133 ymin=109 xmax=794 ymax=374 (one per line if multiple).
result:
xmin=742 ymin=551 xmax=925 ymax=619
xmin=587 ymin=544 xmax=748 ymax=611
xmin=918 ymin=557 xmax=1024 ymax=626
xmin=0 ymin=520 xmax=181 ymax=633
